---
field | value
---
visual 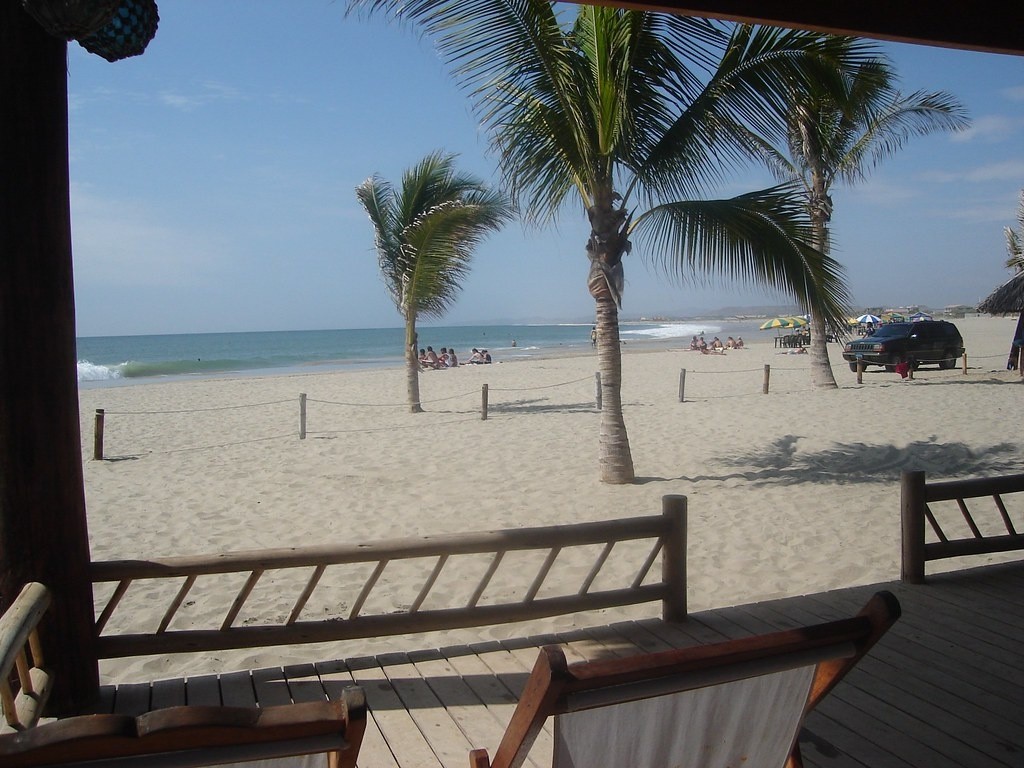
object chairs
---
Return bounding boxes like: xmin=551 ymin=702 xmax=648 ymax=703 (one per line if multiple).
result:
xmin=780 ymin=333 xmax=803 ymax=348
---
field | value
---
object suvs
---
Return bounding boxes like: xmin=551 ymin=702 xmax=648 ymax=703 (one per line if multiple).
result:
xmin=841 ymin=319 xmax=966 ymax=374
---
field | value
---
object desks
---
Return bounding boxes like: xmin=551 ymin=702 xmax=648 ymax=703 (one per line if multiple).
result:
xmin=773 ymin=336 xmax=784 ymax=348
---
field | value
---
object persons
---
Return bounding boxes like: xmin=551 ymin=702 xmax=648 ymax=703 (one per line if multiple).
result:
xmin=590 ymin=327 xmax=597 ymax=346
xmin=736 ymin=336 xmax=743 ymax=346
xmin=726 ymin=336 xmax=736 ymax=347
xmin=690 ymin=335 xmax=708 ymax=354
xmin=459 ymin=348 xmax=491 ymax=365
xmin=803 ymin=327 xmax=806 ymax=331
xmin=713 ymin=337 xmax=722 ymax=348
xmin=418 ymin=346 xmax=458 ymax=369
xmin=877 ymin=319 xmax=882 ymax=328
xmin=795 ymin=327 xmax=799 ymax=335
xmin=863 ymin=322 xmax=875 ymax=340
xmin=512 ymin=340 xmax=516 ymax=347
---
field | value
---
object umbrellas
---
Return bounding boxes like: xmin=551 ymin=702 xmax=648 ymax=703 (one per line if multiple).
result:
xmin=887 ymin=313 xmax=902 ymax=318
xmin=911 ymin=311 xmax=931 ymax=318
xmin=780 ymin=317 xmax=810 ymax=328
xmin=760 ymin=318 xmax=794 ymax=336
xmin=857 ymin=314 xmax=881 ymax=324
